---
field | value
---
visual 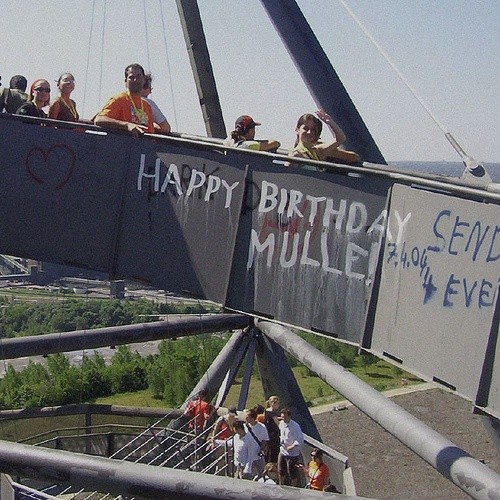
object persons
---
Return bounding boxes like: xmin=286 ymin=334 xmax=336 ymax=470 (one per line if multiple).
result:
xmin=0 ymin=75 xmax=29 ymax=114
xmin=212 ymin=388 xmax=305 ymax=488
xmin=141 ymin=73 xmax=171 ymax=134
xmin=224 ymin=115 xmax=280 ymax=152
xmin=51 ymin=72 xmax=87 ymax=133
xmin=284 ymin=110 xmax=361 ymax=172
xmin=93 ymin=63 xmax=153 ymax=139
xmin=182 ymin=389 xmax=216 ymax=471
xmin=15 ymin=79 xmax=51 ymax=126
xmin=297 ymin=448 xmax=331 ymax=492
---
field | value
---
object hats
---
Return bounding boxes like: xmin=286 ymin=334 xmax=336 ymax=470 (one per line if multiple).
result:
xmin=228 ymin=405 xmax=237 ymax=416
xmin=235 ymin=115 xmax=261 ymax=131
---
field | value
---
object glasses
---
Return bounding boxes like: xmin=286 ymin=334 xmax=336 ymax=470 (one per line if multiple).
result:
xmin=33 ymin=86 xmax=51 ymax=93
xmin=310 ymin=454 xmax=318 ymax=458
